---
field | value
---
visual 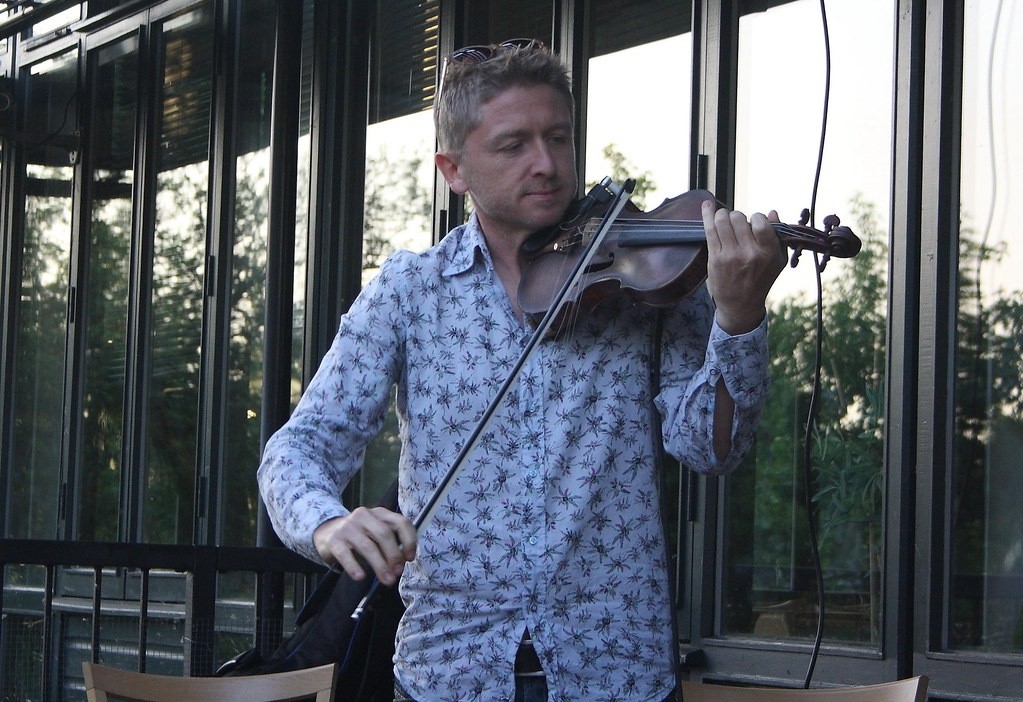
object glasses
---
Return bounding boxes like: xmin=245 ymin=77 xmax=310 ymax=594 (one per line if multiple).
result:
xmin=437 ymin=37 xmax=543 ymax=110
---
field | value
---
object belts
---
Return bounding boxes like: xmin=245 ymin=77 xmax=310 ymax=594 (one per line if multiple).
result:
xmin=515 ymin=639 xmax=545 ymax=678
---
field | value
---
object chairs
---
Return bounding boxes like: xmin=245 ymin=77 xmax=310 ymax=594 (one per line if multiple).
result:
xmin=78 ymin=660 xmax=339 ymax=702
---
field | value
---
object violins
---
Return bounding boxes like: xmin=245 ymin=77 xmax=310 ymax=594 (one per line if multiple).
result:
xmin=514 ymin=188 xmax=860 ymax=329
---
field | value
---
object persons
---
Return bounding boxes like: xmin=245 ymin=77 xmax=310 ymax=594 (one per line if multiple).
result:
xmin=255 ymin=39 xmax=790 ymax=702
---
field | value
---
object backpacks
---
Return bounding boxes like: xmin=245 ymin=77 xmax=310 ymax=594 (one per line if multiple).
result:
xmin=245 ymin=478 xmax=406 ymax=701
